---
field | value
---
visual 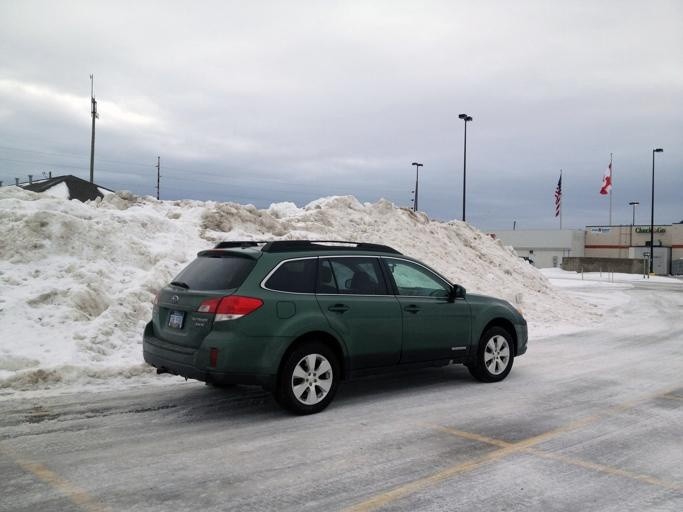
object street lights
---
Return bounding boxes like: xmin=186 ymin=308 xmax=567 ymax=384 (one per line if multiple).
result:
xmin=650 ymin=148 xmax=664 ymax=273
xmin=459 ymin=113 xmax=473 ymax=221
xmin=411 ymin=162 xmax=423 ymax=211
xmin=630 ymin=201 xmax=641 ymax=225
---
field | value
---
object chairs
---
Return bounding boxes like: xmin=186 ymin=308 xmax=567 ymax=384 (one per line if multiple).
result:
xmin=322 ymin=266 xmax=377 ymax=294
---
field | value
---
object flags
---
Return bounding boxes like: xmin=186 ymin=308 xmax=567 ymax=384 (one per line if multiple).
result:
xmin=553 ymin=175 xmax=562 ymax=217
xmin=600 ymin=161 xmax=613 ymax=195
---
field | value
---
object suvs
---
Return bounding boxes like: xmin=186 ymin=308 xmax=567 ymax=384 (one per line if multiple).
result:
xmin=141 ymin=240 xmax=530 ymax=418
